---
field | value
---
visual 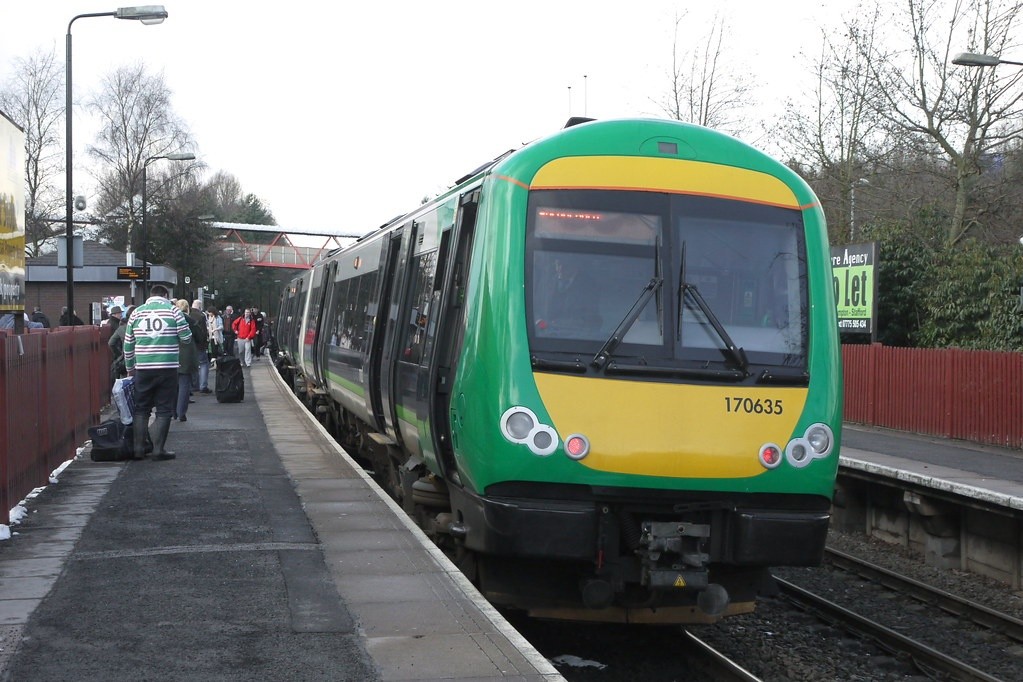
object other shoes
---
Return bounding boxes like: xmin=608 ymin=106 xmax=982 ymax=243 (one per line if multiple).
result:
xmin=200 ymin=388 xmax=212 ymax=393
xmin=189 ymin=391 xmax=193 ymax=396
xmin=178 ymin=415 xmax=186 ymax=421
xmin=188 ymin=399 xmax=195 ymax=403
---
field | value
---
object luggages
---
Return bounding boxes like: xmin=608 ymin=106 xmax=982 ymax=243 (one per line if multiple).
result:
xmin=215 ymin=356 xmax=244 ymax=403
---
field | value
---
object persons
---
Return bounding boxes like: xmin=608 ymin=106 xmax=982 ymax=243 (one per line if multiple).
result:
xmin=251 ymin=306 xmax=271 ymax=361
xmin=204 ymin=306 xmax=224 ymax=367
xmin=189 ymin=299 xmax=213 ymax=395
xmin=304 ymin=323 xmax=315 ymax=361
xmin=330 ymin=327 xmax=366 ymax=352
xmin=547 ymin=252 xmax=602 ymax=338
xmin=232 ymin=309 xmax=256 ymax=368
xmin=101 ymin=305 xmax=139 ymax=379
xmin=221 ymin=306 xmax=240 ymax=357
xmin=124 ymin=286 xmax=203 ymax=461
xmin=32 ymin=307 xmax=50 ymax=329
xmin=59 ymin=307 xmax=84 ymax=326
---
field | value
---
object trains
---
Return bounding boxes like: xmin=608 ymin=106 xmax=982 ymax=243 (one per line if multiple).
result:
xmin=272 ymin=116 xmax=845 ymax=624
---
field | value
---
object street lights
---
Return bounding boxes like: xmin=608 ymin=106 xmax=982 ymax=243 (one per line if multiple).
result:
xmin=180 ymin=214 xmax=216 ymax=300
xmin=848 ymin=178 xmax=871 ymax=243
xmin=142 ymin=152 xmax=196 ymax=305
xmin=64 ymin=4 xmax=170 ymax=322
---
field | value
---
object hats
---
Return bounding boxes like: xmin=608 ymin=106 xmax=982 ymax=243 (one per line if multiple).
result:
xmin=110 ymin=306 xmax=124 ymax=315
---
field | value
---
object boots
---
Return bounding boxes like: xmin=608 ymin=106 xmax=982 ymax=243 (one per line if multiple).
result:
xmin=132 ymin=415 xmax=148 ymax=459
xmin=152 ymin=416 xmax=176 ymax=460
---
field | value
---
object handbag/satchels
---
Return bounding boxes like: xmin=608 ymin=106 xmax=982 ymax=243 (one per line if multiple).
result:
xmin=112 ymin=375 xmax=137 ymax=425
xmin=87 ymin=420 xmax=153 ymax=461
xmin=111 ymin=354 xmax=125 ymax=372
